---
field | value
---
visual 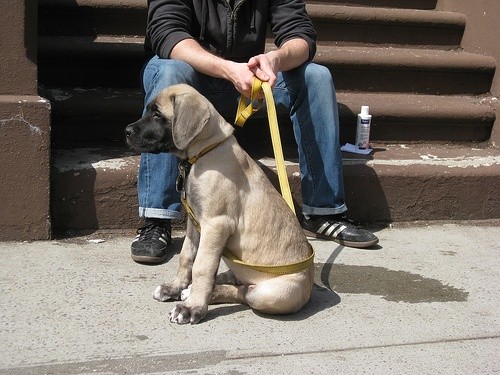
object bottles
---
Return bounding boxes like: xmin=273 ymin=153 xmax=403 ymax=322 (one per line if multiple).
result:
xmin=356 ymin=106 xmax=372 ymax=148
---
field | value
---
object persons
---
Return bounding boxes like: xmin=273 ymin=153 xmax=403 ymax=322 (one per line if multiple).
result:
xmin=130 ymin=0 xmax=379 ymax=261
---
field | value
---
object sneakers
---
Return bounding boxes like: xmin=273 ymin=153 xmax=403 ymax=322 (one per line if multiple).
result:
xmin=130 ymin=218 xmax=173 ymax=263
xmin=297 ymin=215 xmax=379 ymax=248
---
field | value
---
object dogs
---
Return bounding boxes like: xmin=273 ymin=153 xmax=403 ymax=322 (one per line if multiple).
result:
xmin=124 ymin=81 xmax=316 ymax=326
